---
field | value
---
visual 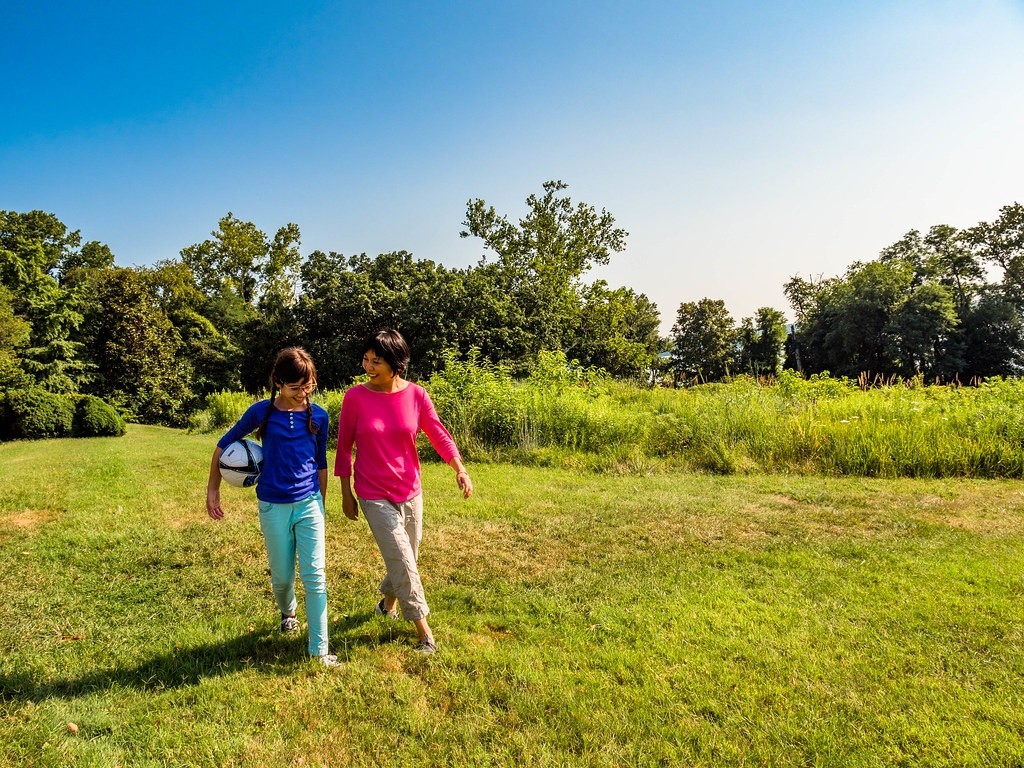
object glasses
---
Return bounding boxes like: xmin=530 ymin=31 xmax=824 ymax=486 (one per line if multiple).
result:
xmin=278 ymin=377 xmax=317 ymax=395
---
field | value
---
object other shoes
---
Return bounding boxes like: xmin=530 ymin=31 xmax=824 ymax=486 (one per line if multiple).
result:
xmin=316 ymin=654 xmax=341 ymax=667
xmin=375 ymin=598 xmax=397 ymax=620
xmin=416 ymin=633 xmax=437 ymax=651
xmin=280 ymin=612 xmax=300 ymax=633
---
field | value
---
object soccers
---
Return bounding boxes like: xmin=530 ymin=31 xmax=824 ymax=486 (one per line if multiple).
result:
xmin=218 ymin=439 xmax=264 ymax=488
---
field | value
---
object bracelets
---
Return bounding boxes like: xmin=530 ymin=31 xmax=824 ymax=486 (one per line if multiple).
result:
xmin=457 ymin=471 xmax=468 ymax=475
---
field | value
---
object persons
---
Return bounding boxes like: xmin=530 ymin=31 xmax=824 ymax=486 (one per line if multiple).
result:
xmin=207 ymin=347 xmax=342 ymax=668
xmin=334 ymin=326 xmax=472 ymax=654
